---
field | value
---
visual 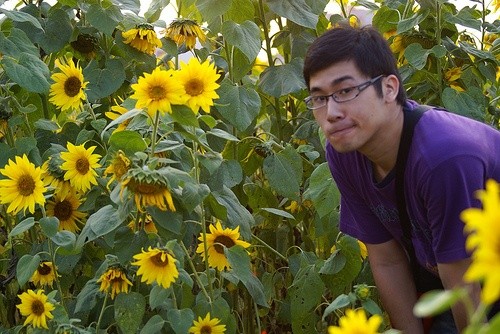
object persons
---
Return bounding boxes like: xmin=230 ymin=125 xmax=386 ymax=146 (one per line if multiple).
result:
xmin=303 ymin=25 xmax=500 ymax=334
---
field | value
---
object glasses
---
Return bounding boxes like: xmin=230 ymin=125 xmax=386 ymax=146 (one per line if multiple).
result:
xmin=304 ymin=74 xmax=386 ymax=112
